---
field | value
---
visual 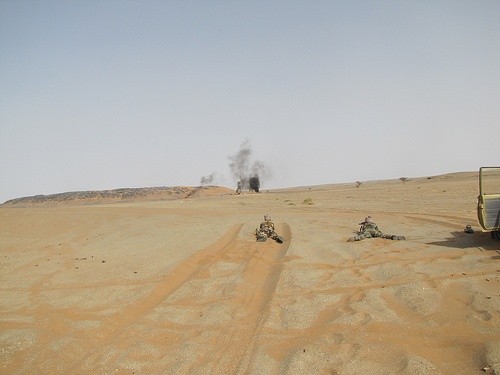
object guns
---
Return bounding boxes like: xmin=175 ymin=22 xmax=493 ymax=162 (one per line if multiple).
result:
xmin=359 ymin=222 xmax=366 ymax=231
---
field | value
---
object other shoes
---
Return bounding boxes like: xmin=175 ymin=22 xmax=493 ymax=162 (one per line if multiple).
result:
xmin=346 ymin=236 xmax=356 ymax=242
xmin=391 ymin=235 xmax=406 ymax=240
xmin=256 ymin=238 xmax=267 ymax=242
xmin=276 ymin=237 xmax=285 ymax=244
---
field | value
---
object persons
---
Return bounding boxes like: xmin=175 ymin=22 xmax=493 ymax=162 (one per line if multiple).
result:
xmin=347 ymin=216 xmax=406 ymax=242
xmin=256 ymin=214 xmax=285 ymax=244
